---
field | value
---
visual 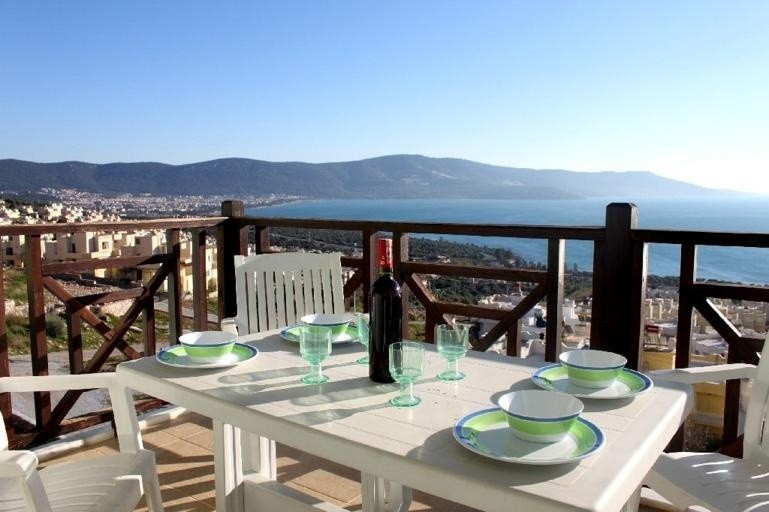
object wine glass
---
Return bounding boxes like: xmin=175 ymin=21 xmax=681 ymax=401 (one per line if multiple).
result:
xmin=437 ymin=322 xmax=470 ymax=381
xmin=387 ymin=340 xmax=425 ymax=410
xmin=297 ymin=325 xmax=332 ymax=384
xmin=357 ymin=314 xmax=370 ymax=365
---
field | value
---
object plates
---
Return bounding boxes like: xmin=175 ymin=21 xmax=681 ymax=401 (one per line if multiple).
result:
xmin=156 ymin=343 xmax=260 ymax=371
xmin=530 ymin=363 xmax=654 ymax=401
xmin=278 ymin=323 xmax=359 ymax=345
xmin=451 ymin=406 xmax=606 ymax=467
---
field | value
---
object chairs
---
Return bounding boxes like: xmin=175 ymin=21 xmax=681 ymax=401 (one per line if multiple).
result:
xmin=0 ymin=372 xmax=164 ymax=512
xmin=219 ymin=251 xmax=344 ymax=340
xmin=639 ymin=334 xmax=769 ymax=512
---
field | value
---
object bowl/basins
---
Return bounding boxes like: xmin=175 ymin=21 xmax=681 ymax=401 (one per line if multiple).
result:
xmin=558 ymin=349 xmax=629 ymax=390
xmin=300 ymin=313 xmax=352 ymax=338
xmin=497 ymin=388 xmax=584 ymax=444
xmin=179 ymin=330 xmax=238 ymax=362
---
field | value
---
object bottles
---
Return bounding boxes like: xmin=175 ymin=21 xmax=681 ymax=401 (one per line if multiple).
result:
xmin=369 ymin=236 xmax=404 ymax=383
